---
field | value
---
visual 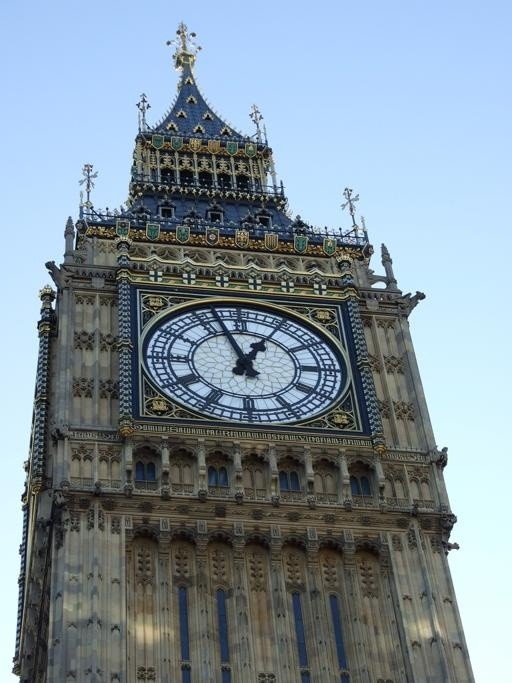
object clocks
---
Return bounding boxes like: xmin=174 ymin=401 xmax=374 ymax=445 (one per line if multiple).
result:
xmin=140 ymin=301 xmax=357 ymax=425
